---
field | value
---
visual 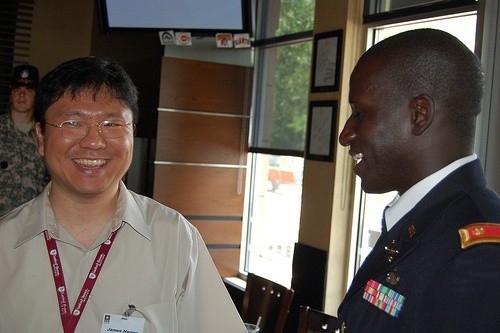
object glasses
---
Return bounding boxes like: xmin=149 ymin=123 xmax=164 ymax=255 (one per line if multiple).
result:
xmin=44 ymin=120 xmax=134 ymax=139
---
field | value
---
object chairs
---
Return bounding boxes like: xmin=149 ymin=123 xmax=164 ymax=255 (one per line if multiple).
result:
xmin=241 ymin=272 xmax=346 ymax=333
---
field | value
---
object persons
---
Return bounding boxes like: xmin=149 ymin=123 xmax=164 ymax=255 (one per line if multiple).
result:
xmin=0 ymin=63 xmax=53 ymax=216
xmin=336 ymin=28 xmax=500 ymax=333
xmin=0 ymin=56 xmax=248 ymax=333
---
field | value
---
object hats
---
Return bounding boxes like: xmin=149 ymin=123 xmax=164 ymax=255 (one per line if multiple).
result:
xmin=11 ymin=64 xmax=39 ymax=85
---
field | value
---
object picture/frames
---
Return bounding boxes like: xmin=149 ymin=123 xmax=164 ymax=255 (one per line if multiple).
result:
xmin=310 ymin=29 xmax=343 ymax=93
xmin=306 ymin=100 xmax=338 ymax=162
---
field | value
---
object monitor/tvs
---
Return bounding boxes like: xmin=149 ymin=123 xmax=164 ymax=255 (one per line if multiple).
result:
xmin=96 ymin=0 xmax=254 ymax=39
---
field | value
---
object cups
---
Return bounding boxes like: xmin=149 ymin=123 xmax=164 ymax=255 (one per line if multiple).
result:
xmin=245 ymin=323 xmax=260 ymax=333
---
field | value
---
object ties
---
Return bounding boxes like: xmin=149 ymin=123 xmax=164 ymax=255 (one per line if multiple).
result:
xmin=380 ymin=206 xmax=387 ymax=237
